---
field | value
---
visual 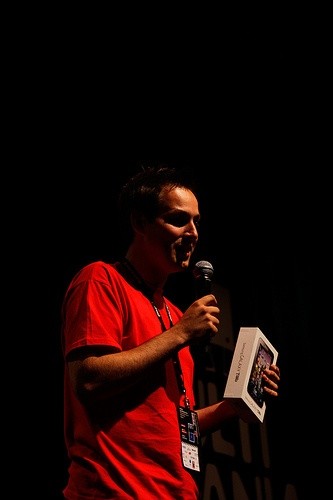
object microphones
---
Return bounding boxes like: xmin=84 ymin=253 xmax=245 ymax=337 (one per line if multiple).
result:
xmin=192 ymin=260 xmax=214 ymax=347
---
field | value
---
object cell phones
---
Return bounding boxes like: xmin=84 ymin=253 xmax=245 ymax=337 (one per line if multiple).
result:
xmin=247 ymin=338 xmax=274 ymax=408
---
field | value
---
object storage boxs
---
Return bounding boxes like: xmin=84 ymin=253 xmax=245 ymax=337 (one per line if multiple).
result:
xmin=224 ymin=327 xmax=279 ymax=423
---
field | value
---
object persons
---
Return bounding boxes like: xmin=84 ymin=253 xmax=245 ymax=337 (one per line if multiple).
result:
xmin=61 ymin=165 xmax=282 ymax=500
